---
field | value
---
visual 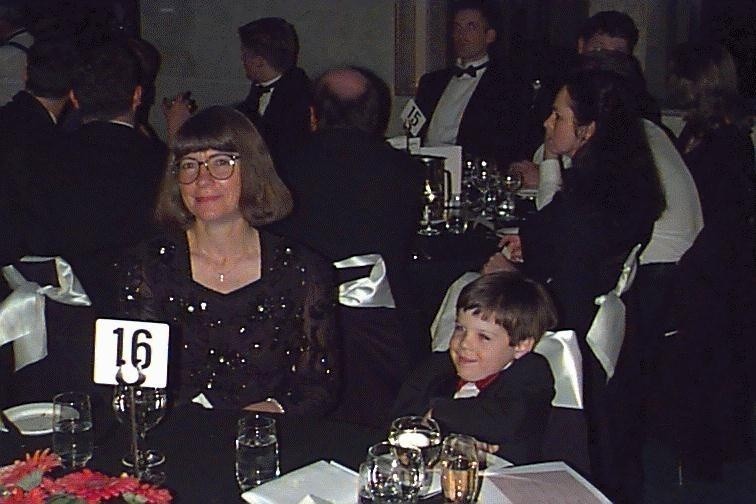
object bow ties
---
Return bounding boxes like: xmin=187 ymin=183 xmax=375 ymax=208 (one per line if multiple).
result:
xmin=455 ymin=59 xmax=490 ymax=77
xmin=253 ymin=78 xmax=280 ymax=95
xmin=455 ymin=374 xmax=499 ymax=391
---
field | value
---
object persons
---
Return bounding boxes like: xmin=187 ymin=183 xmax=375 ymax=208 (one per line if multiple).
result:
xmin=407 ymin=0 xmax=535 ymax=174
xmin=389 ymin=269 xmax=556 ymax=468
xmin=479 ymin=70 xmax=670 ymax=361
xmin=0 ymin=36 xmax=79 ymax=266
xmin=264 ymin=64 xmax=428 ymax=261
xmin=508 ymin=48 xmax=706 ymax=320
xmin=109 ymin=105 xmax=346 ymax=422
xmin=31 ymin=35 xmax=166 ymax=316
xmin=576 ymin=8 xmax=640 ymax=55
xmin=237 ymin=16 xmax=316 ymax=178
xmin=665 ymin=37 xmax=756 ymax=240
xmin=60 ymin=36 xmax=195 ymax=222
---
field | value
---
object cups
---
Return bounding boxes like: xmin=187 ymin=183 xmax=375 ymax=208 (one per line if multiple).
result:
xmin=51 ymin=433 xmax=94 ymax=463
xmin=441 ymin=432 xmax=480 ymax=503
xmin=56 ymin=390 xmax=93 ymax=436
xmin=236 ymin=416 xmax=281 ymax=490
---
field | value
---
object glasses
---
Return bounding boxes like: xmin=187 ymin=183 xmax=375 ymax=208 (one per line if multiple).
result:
xmin=173 ymin=153 xmax=243 ymax=184
xmin=445 ymin=21 xmax=484 ymax=40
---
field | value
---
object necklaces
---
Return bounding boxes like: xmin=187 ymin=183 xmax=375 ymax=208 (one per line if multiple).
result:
xmin=197 ymin=243 xmax=246 ymax=283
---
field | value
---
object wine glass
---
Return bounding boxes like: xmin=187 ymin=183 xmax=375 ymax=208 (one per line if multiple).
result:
xmin=495 ymin=170 xmax=523 ymax=223
xmin=358 ymin=459 xmax=404 ymax=502
xmin=366 ymin=442 xmax=426 ymax=503
xmin=391 ymin=465 xmax=436 ymax=497
xmin=114 ymin=387 xmax=168 ymax=468
xmin=389 ymin=415 xmax=442 ymax=470
xmin=470 ymin=154 xmax=504 ymax=221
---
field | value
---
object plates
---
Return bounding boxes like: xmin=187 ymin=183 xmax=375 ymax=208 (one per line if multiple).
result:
xmin=2 ymin=401 xmax=79 ymax=436
